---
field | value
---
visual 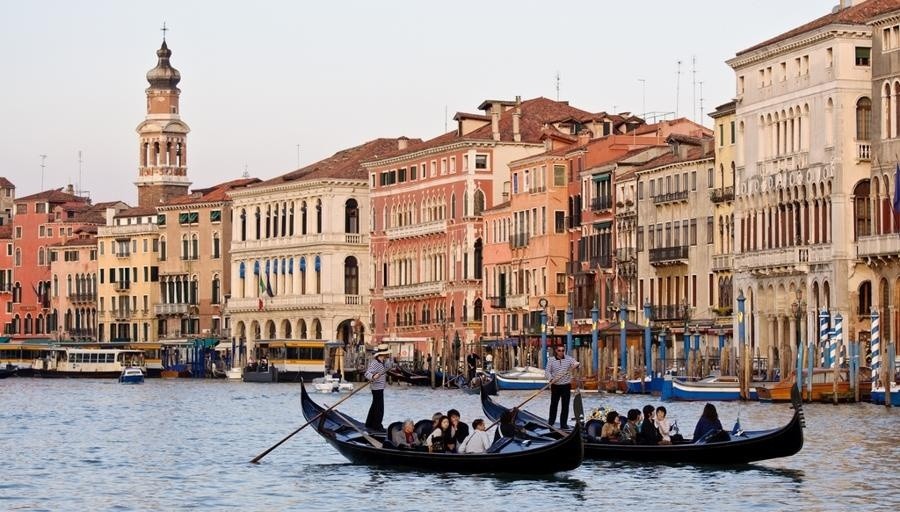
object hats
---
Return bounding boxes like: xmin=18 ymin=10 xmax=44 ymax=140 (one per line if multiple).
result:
xmin=374 ymin=344 xmax=392 ymax=358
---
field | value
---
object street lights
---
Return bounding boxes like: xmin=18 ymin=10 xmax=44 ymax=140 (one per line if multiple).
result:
xmin=547 ymin=305 xmax=559 ymax=348
xmin=678 ymin=295 xmax=692 ymax=332
xmin=790 ymin=290 xmax=807 ymax=348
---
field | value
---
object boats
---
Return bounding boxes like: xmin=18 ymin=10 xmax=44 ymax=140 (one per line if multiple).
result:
xmin=388 ymin=354 xmax=548 ymax=390
xmin=466 ymin=368 xmax=806 ymax=468
xmin=241 ymin=353 xmax=280 ymax=384
xmin=672 ymin=376 xmax=781 ymax=401
xmin=0 ymin=338 xmax=247 ymax=376
xmin=309 ymin=344 xmax=359 ymax=397
xmin=296 ymin=383 xmax=586 ymax=475
xmin=117 ymin=367 xmax=147 ymax=383
xmin=624 ymin=366 xmax=685 ymax=394
xmin=755 ymin=366 xmax=874 ymax=402
xmin=870 ymin=380 xmax=900 ymax=406
xmin=252 ymin=338 xmax=360 ymax=382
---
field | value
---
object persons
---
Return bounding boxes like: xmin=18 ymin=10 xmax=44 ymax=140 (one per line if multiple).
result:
xmin=486 ymin=351 xmax=494 ymax=370
xmin=467 ymin=354 xmax=480 ymax=383
xmin=601 ymin=405 xmax=671 ymax=445
xmin=436 ymin=354 xmax=440 ymax=368
xmin=38 ymin=358 xmax=51 ymax=370
xmin=365 ymin=344 xmax=401 ymax=432
xmin=394 ymin=408 xmax=491 ymax=454
xmin=6 ymin=361 xmax=18 ymax=371
xmin=694 ymin=403 xmax=722 ymax=443
xmin=545 ymin=344 xmax=581 ymax=429
xmin=427 ymin=353 xmax=432 ymax=371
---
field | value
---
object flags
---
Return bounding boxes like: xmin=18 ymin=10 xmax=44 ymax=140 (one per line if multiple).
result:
xmin=259 ymin=276 xmax=267 ymax=293
xmin=259 ymin=299 xmax=263 ymax=310
xmin=266 ymin=275 xmax=274 ymax=297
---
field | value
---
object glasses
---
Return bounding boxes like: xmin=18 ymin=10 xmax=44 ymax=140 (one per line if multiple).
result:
xmin=557 ymin=350 xmax=565 ymax=352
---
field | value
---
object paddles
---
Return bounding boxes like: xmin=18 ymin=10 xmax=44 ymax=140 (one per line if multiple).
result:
xmin=324 ymin=402 xmax=383 ymax=448
xmin=250 ymin=367 xmax=394 ymax=464
xmin=486 ymin=365 xmax=575 ymax=431
